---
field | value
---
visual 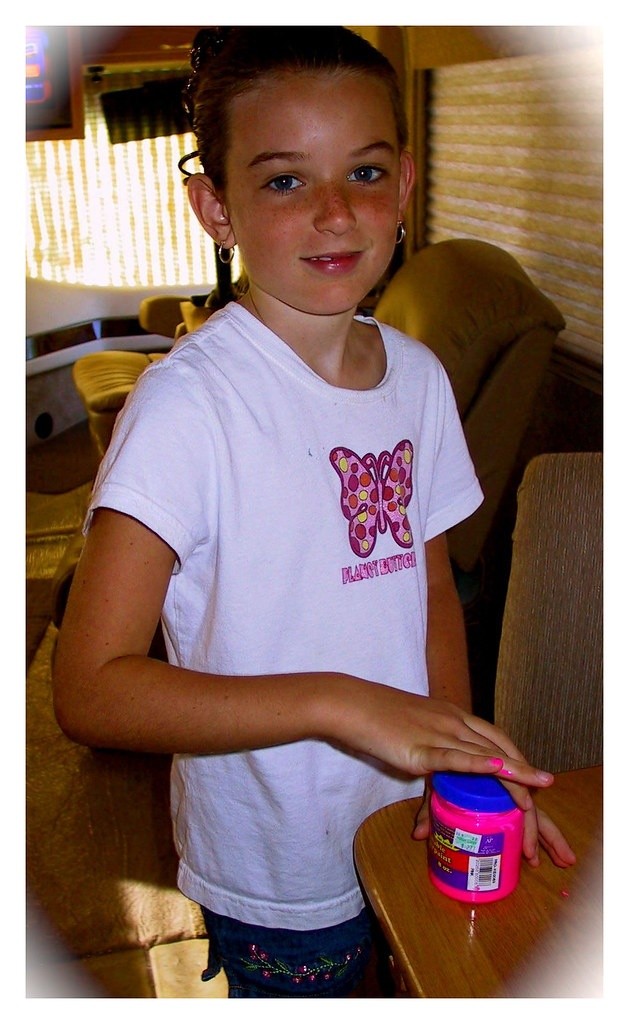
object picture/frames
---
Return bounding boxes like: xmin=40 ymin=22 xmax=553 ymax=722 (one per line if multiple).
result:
xmin=26 ymin=26 xmax=87 ymax=142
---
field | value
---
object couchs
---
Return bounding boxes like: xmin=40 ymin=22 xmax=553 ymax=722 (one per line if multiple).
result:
xmin=52 ymin=239 xmax=565 ymax=631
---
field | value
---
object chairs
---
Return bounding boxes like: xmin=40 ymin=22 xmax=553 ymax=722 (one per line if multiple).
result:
xmin=492 ymin=453 xmax=603 ymax=772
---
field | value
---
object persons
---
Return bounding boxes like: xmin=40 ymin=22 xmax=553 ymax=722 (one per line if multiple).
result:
xmin=53 ymin=25 xmax=581 ymax=998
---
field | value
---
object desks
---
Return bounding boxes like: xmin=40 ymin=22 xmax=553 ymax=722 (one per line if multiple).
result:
xmin=352 ymin=767 xmax=603 ymax=998
